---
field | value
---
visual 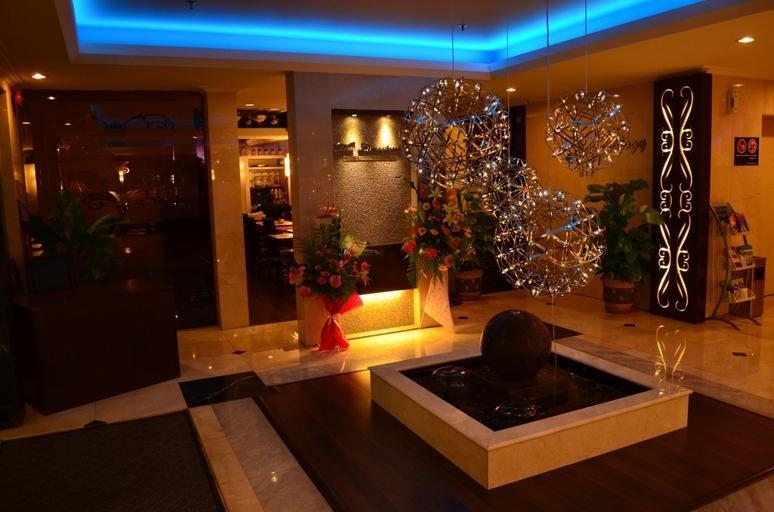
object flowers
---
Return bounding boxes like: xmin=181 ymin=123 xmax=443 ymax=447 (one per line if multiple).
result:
xmin=288 ymin=201 xmax=381 ymax=303
xmin=400 ymin=177 xmax=495 ymax=288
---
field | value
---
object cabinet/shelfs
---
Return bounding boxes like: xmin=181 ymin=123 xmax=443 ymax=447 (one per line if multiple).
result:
xmin=706 ymin=202 xmax=763 ymax=331
xmin=239 ymin=154 xmax=292 ymax=212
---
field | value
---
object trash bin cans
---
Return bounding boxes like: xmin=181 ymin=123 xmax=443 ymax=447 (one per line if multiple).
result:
xmin=729 ymin=255 xmax=766 ymax=318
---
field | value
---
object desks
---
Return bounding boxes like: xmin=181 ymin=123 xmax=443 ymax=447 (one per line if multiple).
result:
xmin=12 ymin=277 xmax=181 ymax=415
xmin=256 ymin=220 xmax=293 ymax=233
xmin=266 ymin=232 xmax=294 ymax=287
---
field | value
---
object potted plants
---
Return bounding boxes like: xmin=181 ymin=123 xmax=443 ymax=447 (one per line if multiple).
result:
xmin=568 ymin=179 xmax=664 ymax=313
xmin=17 ymin=186 xmax=130 ymax=298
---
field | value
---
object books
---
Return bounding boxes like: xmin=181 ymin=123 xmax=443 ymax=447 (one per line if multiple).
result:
xmin=730 ymin=278 xmax=748 ymax=300
xmin=728 ymin=245 xmax=753 ymax=267
xmin=728 ymin=213 xmax=750 ymax=233
